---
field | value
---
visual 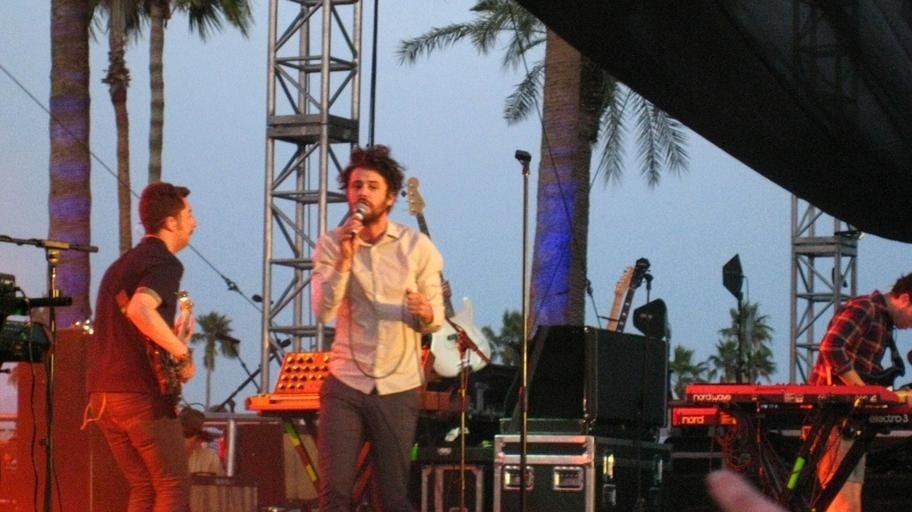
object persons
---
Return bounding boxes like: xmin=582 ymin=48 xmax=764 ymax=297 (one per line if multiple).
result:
xmin=801 ymin=272 xmax=912 ymax=512
xmin=707 ymin=470 xmax=788 ymax=512
xmin=311 ymin=150 xmax=445 ymax=512
xmin=86 ymin=183 xmax=197 ymax=512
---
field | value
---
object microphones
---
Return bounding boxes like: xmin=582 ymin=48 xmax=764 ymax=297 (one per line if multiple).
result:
xmin=350 ymin=202 xmax=368 ymax=241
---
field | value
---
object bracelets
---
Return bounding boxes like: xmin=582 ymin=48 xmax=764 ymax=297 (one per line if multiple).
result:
xmin=179 ymin=348 xmax=192 ymax=366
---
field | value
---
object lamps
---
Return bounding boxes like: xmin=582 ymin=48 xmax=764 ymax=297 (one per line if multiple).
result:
xmin=634 ymin=299 xmax=672 ymax=340
xmin=722 ymin=253 xmax=746 ymax=300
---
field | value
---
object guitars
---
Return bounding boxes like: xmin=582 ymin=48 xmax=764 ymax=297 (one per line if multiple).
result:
xmin=147 ymin=290 xmax=194 ymax=396
xmin=407 ymin=178 xmax=492 ymax=378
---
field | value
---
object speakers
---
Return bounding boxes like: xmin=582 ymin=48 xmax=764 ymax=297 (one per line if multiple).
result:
xmin=527 ymin=326 xmax=668 ymax=429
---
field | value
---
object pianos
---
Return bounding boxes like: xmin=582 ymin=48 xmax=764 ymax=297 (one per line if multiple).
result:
xmin=668 ymin=384 xmax=912 ymax=435
